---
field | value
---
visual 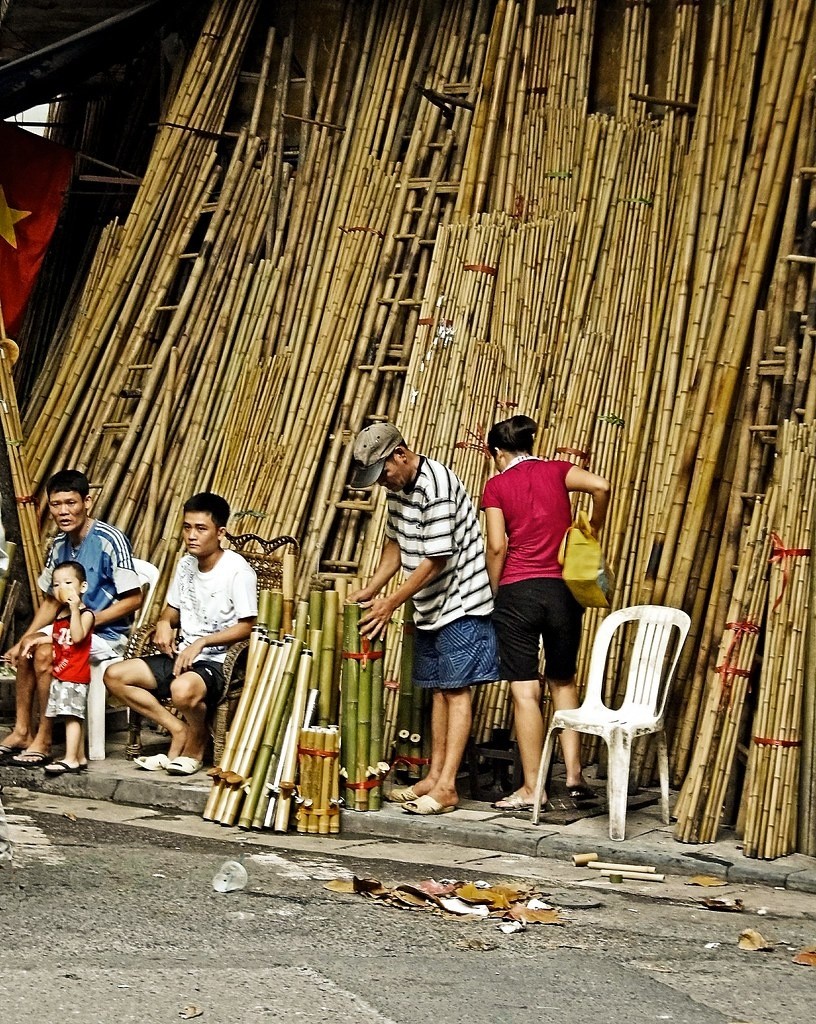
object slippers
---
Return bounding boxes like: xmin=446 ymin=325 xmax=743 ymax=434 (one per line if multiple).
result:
xmin=0 ymin=745 xmax=27 ymax=759
xmin=491 ymin=792 xmax=548 ymax=813
xmin=165 ymin=756 xmax=204 ymax=774
xmin=133 ymin=753 xmax=170 ymax=771
xmin=44 ymin=762 xmax=80 ymax=773
xmin=402 ymin=795 xmax=455 ymax=815
xmin=569 ymin=783 xmax=596 ymax=798
xmin=386 ymin=785 xmax=419 ymax=803
xmin=80 ymin=764 xmax=88 ymax=770
xmin=11 ymin=750 xmax=53 ymax=765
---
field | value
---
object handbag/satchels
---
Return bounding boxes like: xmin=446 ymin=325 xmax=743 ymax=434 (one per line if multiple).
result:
xmin=556 ymin=511 xmax=616 ymax=609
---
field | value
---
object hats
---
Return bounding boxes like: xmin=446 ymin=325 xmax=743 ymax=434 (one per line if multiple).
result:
xmin=350 ymin=422 xmax=403 ymax=488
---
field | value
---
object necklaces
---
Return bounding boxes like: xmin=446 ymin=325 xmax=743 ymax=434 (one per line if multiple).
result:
xmin=70 ymin=517 xmax=90 ymax=559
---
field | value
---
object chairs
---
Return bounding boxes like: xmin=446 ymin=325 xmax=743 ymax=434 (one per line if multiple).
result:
xmin=122 ymin=534 xmax=299 ymax=768
xmin=106 ymin=558 xmax=160 ymax=761
xmin=529 ymin=606 xmax=690 ymax=838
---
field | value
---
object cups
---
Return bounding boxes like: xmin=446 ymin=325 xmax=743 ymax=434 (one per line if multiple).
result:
xmin=212 ymin=860 xmax=248 ymax=892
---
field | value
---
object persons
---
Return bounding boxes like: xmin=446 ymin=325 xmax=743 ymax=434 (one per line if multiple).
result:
xmin=0 ymin=471 xmax=142 ymax=767
xmin=103 ymin=492 xmax=258 ymax=774
xmin=477 ymin=415 xmax=611 ymax=809
xmin=345 ymin=423 xmax=502 ymax=815
xmin=21 ymin=561 xmax=96 ymax=772
